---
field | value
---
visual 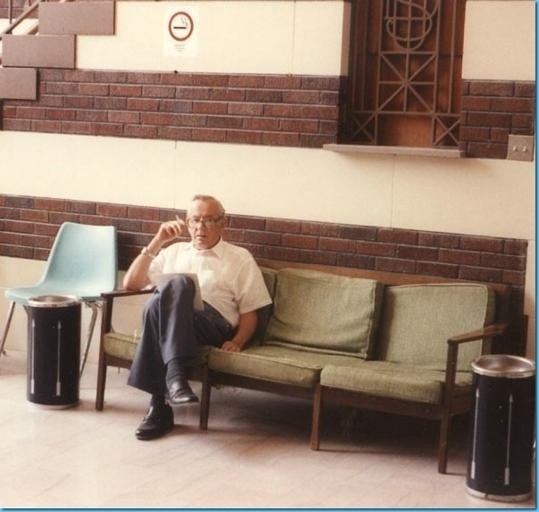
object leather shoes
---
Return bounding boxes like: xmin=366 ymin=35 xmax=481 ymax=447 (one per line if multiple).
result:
xmin=165 ymin=375 xmax=199 ymax=407
xmin=135 ymin=404 xmax=174 ymax=438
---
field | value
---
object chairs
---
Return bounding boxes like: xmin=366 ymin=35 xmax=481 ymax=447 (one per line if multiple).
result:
xmin=0 ymin=221 xmax=124 ymax=381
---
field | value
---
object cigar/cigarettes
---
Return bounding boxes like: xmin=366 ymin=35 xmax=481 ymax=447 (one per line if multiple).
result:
xmin=175 ymin=212 xmax=182 ymax=228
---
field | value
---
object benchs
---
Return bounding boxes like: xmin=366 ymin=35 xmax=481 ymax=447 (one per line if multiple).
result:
xmin=95 ymin=256 xmax=518 ymax=475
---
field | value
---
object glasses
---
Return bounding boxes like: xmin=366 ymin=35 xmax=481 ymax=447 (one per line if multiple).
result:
xmin=186 ymin=216 xmax=221 ymax=229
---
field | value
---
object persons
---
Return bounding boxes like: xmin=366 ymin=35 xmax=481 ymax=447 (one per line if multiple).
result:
xmin=122 ymin=191 xmax=273 ymax=441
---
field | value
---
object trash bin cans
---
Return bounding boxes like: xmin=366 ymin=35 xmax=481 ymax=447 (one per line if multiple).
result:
xmin=27 ymin=295 xmax=81 ymax=410
xmin=465 ymin=354 xmax=536 ymax=502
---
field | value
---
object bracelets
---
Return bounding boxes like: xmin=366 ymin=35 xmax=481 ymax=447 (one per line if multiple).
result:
xmin=141 ymin=245 xmax=159 ymax=259
